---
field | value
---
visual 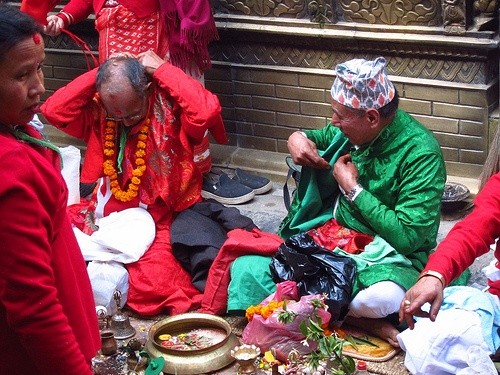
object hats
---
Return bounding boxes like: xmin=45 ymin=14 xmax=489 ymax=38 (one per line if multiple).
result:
xmin=330 ymin=57 xmax=395 ymax=109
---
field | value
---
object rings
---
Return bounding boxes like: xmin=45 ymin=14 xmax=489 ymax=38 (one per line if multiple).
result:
xmin=404 ymin=300 xmax=411 ymax=305
xmin=116 ymin=53 xmax=122 ymax=57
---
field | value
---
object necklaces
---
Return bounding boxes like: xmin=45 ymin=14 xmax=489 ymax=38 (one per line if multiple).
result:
xmin=102 ymin=114 xmax=151 ymax=203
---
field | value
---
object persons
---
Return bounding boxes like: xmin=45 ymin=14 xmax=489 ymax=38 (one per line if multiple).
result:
xmin=0 ymin=3 xmax=101 ymax=375
xmin=398 ymin=172 xmax=500 ymax=375
xmin=35 ymin=49 xmax=229 ymax=316
xmin=225 ymin=56 xmax=448 ymax=331
xmin=39 ymin=0 xmax=221 ymax=199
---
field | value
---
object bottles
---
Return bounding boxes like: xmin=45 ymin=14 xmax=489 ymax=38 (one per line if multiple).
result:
xmin=355 ymin=362 xmax=371 ymax=374
xmin=100 ymin=330 xmax=118 ymax=356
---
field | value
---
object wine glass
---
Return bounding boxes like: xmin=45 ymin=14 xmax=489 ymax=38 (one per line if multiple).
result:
xmin=230 ymin=344 xmax=263 ymax=375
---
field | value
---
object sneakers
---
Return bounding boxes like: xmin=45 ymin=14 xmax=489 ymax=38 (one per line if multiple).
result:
xmin=203 ymin=166 xmax=272 ymax=205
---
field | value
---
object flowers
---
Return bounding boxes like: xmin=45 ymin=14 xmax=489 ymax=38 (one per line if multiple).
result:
xmin=243 ymin=291 xmax=380 ymax=375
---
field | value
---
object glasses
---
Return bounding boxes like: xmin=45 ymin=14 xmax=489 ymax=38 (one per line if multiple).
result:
xmin=104 ymin=95 xmax=146 ymax=122
